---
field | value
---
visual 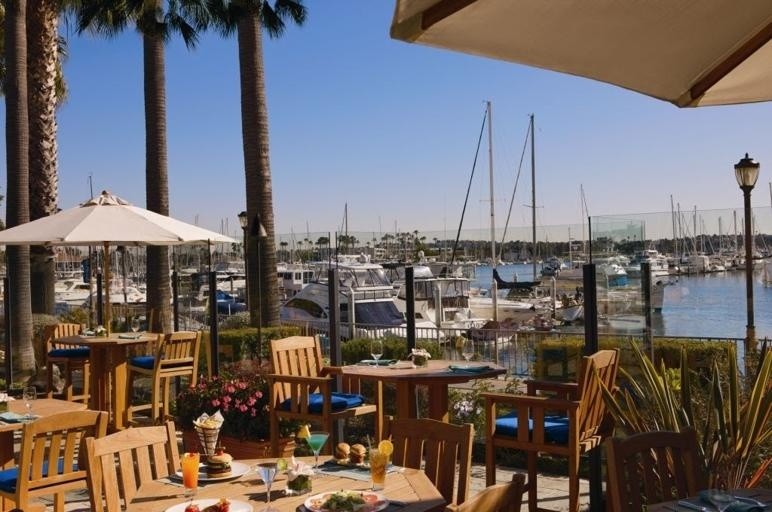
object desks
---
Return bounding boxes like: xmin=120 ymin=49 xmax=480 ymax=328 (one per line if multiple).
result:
xmin=0 ymin=398 xmax=87 ymax=470
xmin=127 ymin=454 xmax=448 ymax=511
xmin=50 ymin=332 xmax=159 ymax=431
xmin=340 ymin=358 xmax=508 ymax=420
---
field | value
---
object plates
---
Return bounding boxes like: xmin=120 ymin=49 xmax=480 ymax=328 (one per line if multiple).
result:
xmin=176 ymin=460 xmax=250 ymax=480
xmin=166 ymin=497 xmax=253 ymax=512
xmin=303 ymin=490 xmax=390 ymax=511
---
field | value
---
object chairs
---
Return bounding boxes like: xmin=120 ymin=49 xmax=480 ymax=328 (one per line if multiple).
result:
xmin=382 ymin=420 xmax=526 ymax=512
xmin=264 ymin=332 xmax=383 ymax=453
xmin=83 ymin=421 xmax=180 ymax=511
xmin=479 ymin=346 xmax=622 ymax=511
xmin=1 ymin=409 xmax=109 ymax=512
xmin=41 ymin=321 xmax=91 ymax=406
xmin=126 ymin=330 xmax=204 ymax=425
xmin=605 ymin=425 xmax=710 ymax=512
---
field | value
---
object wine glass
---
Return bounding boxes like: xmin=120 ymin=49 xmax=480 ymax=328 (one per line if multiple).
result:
xmin=22 ymin=386 xmax=37 ymax=421
xmin=252 ymin=462 xmax=280 ymax=511
xmin=306 ymin=430 xmax=330 ymax=474
xmin=370 ymin=339 xmax=384 ymax=370
xmin=461 ymin=339 xmax=475 ymax=367
xmin=131 ymin=317 xmax=141 ymax=341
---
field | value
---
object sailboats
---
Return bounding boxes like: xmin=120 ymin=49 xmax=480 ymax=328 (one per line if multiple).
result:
xmin=54 ymin=100 xmax=772 ymax=352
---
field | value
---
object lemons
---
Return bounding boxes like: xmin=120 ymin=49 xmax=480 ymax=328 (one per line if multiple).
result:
xmin=378 ymin=440 xmax=394 ymax=455
xmin=276 ymin=458 xmax=287 ymax=472
xmin=297 ymin=425 xmax=310 ymax=438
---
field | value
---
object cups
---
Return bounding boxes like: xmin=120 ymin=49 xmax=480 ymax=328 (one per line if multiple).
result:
xmin=369 ymin=449 xmax=387 ymax=490
xmin=180 ymin=453 xmax=201 ymax=495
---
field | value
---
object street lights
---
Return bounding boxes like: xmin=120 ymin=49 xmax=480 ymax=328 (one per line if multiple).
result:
xmin=734 ymin=153 xmax=762 ymax=374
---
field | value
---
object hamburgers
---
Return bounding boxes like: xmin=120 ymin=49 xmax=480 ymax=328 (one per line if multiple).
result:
xmin=350 ymin=444 xmax=366 ymax=463
xmin=335 ymin=442 xmax=350 ymax=460
xmin=206 ymin=451 xmax=232 ymax=477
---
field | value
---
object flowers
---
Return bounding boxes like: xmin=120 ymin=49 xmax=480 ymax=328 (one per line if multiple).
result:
xmin=176 ymin=356 xmax=306 ymax=443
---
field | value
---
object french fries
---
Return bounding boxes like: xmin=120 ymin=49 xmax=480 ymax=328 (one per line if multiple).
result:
xmin=194 ymin=418 xmax=219 ymax=430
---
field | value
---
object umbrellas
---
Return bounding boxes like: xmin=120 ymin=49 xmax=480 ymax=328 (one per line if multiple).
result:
xmin=0 ymin=190 xmax=241 ymax=337
xmin=391 ymin=1 xmax=771 ymax=109
